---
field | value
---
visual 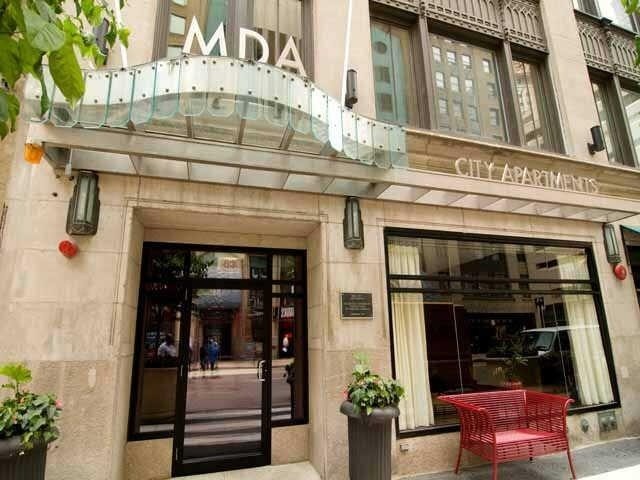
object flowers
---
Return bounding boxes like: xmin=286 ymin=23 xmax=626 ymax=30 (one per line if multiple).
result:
xmin=0 ymin=361 xmax=64 ymax=454
xmin=343 ymin=353 xmax=408 ymax=415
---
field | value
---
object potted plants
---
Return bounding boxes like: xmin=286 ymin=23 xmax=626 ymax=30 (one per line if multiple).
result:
xmin=491 ymin=337 xmax=529 ymax=388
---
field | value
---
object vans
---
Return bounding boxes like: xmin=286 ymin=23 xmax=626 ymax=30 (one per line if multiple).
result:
xmin=145 ymin=332 xmax=174 ymax=365
xmin=518 ymin=325 xmax=603 ymax=381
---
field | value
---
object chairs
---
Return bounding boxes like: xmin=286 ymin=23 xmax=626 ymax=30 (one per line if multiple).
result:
xmin=436 ymin=388 xmax=578 ymax=480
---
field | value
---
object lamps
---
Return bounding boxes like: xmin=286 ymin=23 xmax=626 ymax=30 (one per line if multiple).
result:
xmin=65 ymin=172 xmax=100 ymax=234
xmin=343 ymin=68 xmax=358 ymax=108
xmin=587 ymin=126 xmax=605 ymax=154
xmin=602 ymin=223 xmax=622 ymax=263
xmin=91 ymin=16 xmax=109 ymax=65
xmin=342 ymin=196 xmax=364 ymax=250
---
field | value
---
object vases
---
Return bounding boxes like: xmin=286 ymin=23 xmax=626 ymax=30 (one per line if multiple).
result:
xmin=0 ymin=435 xmax=48 ymax=480
xmin=339 ymin=400 xmax=400 ymax=480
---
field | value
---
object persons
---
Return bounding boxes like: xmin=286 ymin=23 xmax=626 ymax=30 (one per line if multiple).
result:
xmin=207 ymin=338 xmax=221 ymax=369
xmin=198 ymin=341 xmax=207 ymax=371
xmin=281 ymin=334 xmax=291 ymax=357
xmin=156 ymin=332 xmax=177 ymax=366
